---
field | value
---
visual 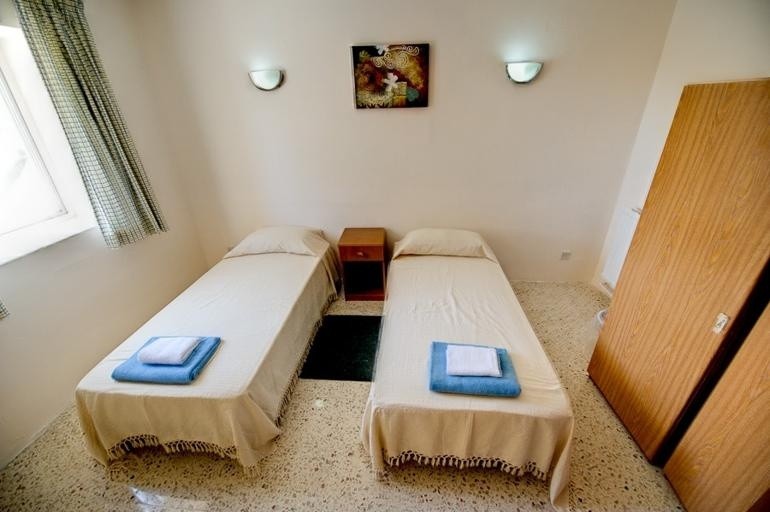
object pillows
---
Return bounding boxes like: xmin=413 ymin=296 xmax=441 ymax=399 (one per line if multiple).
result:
xmin=392 ymin=229 xmax=502 ymax=264
xmin=221 ymin=226 xmax=326 ymax=260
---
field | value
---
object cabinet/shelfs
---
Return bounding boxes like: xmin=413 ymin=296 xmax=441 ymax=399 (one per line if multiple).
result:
xmin=588 ymin=78 xmax=770 ymax=465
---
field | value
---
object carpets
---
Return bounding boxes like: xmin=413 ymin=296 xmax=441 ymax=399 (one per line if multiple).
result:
xmin=298 ymin=314 xmax=384 ymax=382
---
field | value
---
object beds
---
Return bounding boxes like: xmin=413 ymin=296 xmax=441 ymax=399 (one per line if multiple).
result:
xmin=75 ymin=236 xmax=331 ymax=454
xmin=373 ymin=252 xmax=571 ymax=474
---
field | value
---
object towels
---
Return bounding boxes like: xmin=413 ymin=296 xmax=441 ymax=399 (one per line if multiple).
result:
xmin=445 ymin=344 xmax=503 ymax=378
xmin=137 ymin=337 xmax=201 ymax=364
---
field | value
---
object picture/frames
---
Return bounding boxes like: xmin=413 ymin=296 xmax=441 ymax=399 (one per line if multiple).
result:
xmin=349 ymin=41 xmax=431 ymax=111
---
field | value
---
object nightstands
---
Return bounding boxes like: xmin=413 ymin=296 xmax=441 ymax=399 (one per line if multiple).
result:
xmin=338 ymin=227 xmax=386 ymax=303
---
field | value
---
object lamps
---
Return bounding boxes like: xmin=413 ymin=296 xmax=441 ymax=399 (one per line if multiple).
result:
xmin=248 ymin=69 xmax=283 ymax=91
xmin=505 ymin=61 xmax=543 ymax=84
